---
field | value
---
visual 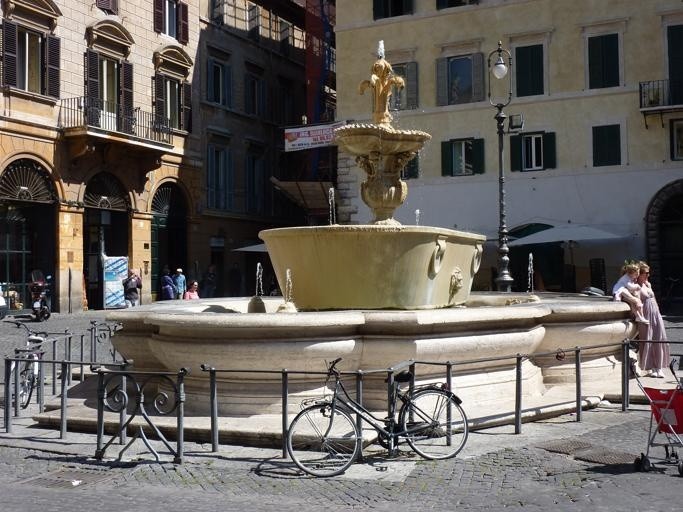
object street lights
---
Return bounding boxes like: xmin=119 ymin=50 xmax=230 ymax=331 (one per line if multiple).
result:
xmin=486 ymin=40 xmax=518 ymax=294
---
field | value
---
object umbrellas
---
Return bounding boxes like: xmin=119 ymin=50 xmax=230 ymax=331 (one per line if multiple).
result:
xmin=508 ymin=217 xmax=638 ymax=262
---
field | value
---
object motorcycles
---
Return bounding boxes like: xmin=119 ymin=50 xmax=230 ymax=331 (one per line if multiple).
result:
xmin=27 ymin=269 xmax=54 ymax=322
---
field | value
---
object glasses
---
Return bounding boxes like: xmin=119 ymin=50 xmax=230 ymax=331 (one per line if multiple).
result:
xmin=642 ymin=272 xmax=649 ymax=274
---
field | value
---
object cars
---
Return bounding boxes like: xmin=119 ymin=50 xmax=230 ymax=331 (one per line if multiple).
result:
xmin=0 ymin=281 xmax=21 ymax=308
xmin=0 ymin=285 xmax=6 ymax=322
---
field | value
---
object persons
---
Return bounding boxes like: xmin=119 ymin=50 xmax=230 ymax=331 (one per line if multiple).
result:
xmin=183 ymin=278 xmax=199 ymax=301
xmin=120 ymin=267 xmax=141 ymax=309
xmin=610 ymin=263 xmax=650 ymax=328
xmin=200 ymin=264 xmax=216 ymax=299
xmin=228 ymin=262 xmax=243 ymax=295
xmin=631 ymin=255 xmax=673 ymax=376
xmin=155 ymin=267 xmax=177 ymax=299
xmin=171 ymin=268 xmax=187 ymax=301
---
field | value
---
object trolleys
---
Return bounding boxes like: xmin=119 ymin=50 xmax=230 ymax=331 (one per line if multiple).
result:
xmin=616 ymin=336 xmax=682 ymax=475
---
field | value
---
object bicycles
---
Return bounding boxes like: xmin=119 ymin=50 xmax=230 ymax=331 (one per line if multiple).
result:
xmin=3 ymin=319 xmax=49 ymax=408
xmin=285 ymin=357 xmax=473 ymax=480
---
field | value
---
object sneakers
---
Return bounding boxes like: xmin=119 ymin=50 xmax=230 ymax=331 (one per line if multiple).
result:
xmin=649 ymin=372 xmax=656 ymax=377
xmin=634 ymin=316 xmax=649 ymax=323
xmin=656 ymin=372 xmax=663 ymax=378
xmin=641 ymin=317 xmax=650 ymax=325
